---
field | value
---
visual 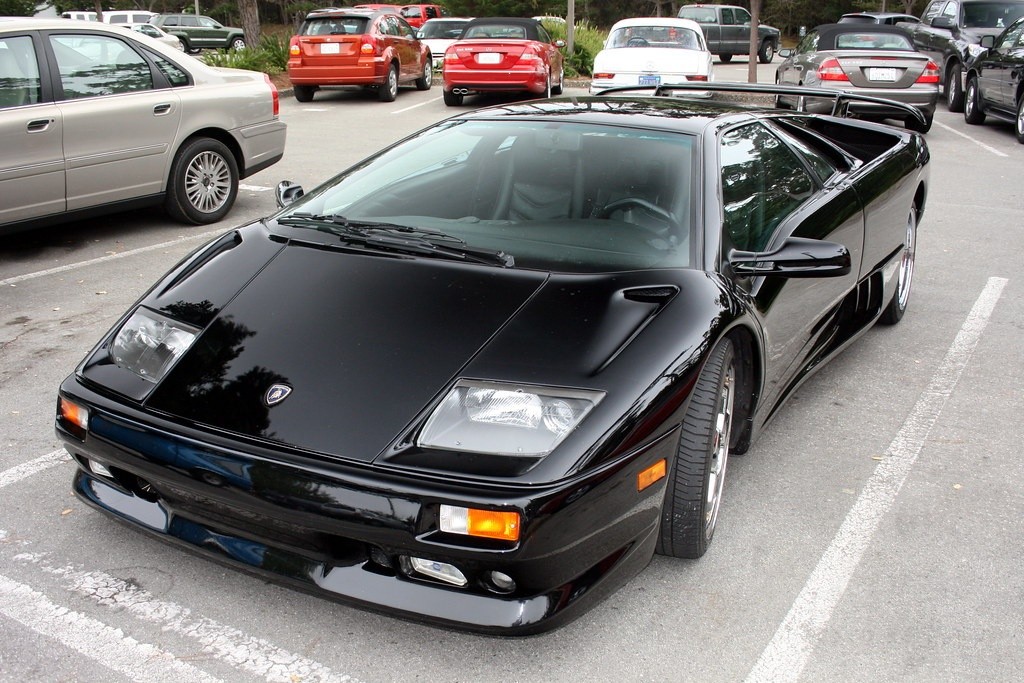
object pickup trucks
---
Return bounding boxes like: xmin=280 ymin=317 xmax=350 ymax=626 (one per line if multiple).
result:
xmin=895 ymin=0 xmax=1024 ymax=112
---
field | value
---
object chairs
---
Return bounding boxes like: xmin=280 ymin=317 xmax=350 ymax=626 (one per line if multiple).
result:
xmin=589 ymin=138 xmax=690 ymax=233
xmin=487 ymin=132 xmax=584 ymax=220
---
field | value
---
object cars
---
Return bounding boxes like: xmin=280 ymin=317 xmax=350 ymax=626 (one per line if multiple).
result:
xmin=589 ymin=17 xmax=714 ymax=99
xmin=963 ymin=15 xmax=1024 ymax=144
xmin=775 ymin=23 xmax=940 ymax=133
xmin=0 ymin=15 xmax=288 ymax=229
xmin=442 ymin=17 xmax=564 ymax=107
xmin=355 ymin=5 xmax=476 ymax=74
xmin=838 ymin=12 xmax=923 ymax=49
xmin=531 ymin=16 xmax=586 ymax=31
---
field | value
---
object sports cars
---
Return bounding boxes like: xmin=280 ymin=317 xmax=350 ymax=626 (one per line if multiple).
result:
xmin=56 ymin=83 xmax=930 ymax=638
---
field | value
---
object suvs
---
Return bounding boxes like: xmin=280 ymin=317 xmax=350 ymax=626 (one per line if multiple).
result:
xmin=285 ymin=9 xmax=433 ymax=103
xmin=62 ymin=10 xmax=246 ymax=54
xmin=677 ymin=5 xmax=783 ymax=63
